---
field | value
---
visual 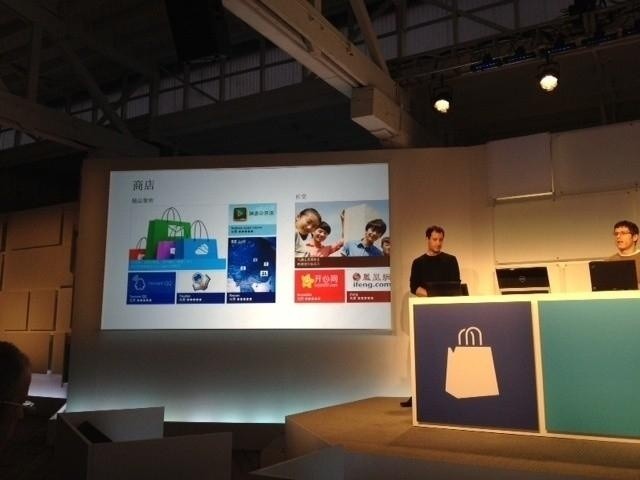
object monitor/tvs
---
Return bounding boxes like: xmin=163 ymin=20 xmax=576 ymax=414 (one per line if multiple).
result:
xmin=496 ymin=267 xmax=550 ymax=295
xmin=588 ymin=260 xmax=638 ymax=291
xmin=423 ymin=282 xmax=469 ymax=296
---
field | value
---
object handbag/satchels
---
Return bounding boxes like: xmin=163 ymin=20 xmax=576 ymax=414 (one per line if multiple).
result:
xmin=156 ymin=241 xmax=175 ymax=260
xmin=174 ymin=236 xmax=216 ymax=261
xmin=129 ymin=249 xmax=146 ymax=260
xmin=444 ymin=345 xmax=500 ymax=401
xmin=143 ymin=219 xmax=191 ymax=260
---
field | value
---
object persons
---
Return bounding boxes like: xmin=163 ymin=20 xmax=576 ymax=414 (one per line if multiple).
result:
xmin=340 ymin=217 xmax=387 ymax=257
xmin=1 ymin=339 xmax=34 ymax=480
xmin=609 ymin=220 xmax=640 ymax=290
xmin=381 ymin=236 xmax=390 ymax=256
xmin=295 ymin=206 xmax=321 ymax=257
xmin=307 ymin=209 xmax=346 ymax=257
xmin=400 ymin=225 xmax=461 ymax=407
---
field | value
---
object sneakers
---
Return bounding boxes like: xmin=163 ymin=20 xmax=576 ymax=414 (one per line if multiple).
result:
xmin=400 ymin=397 xmax=412 ymax=407
